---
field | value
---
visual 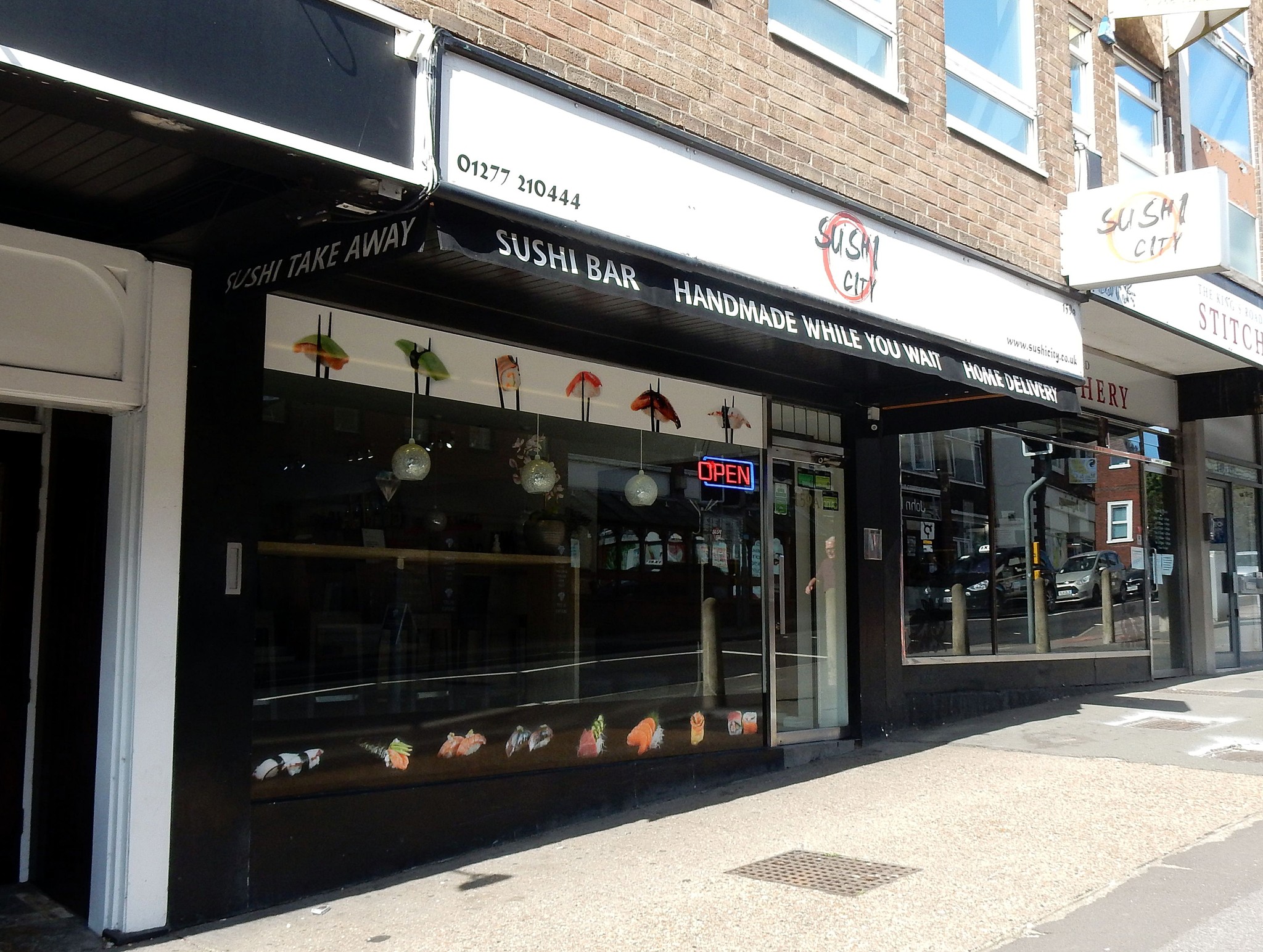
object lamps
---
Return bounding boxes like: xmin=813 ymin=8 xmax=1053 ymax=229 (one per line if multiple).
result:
xmin=624 ymin=429 xmax=658 ymax=508
xmin=390 ymin=392 xmax=431 ymax=481
xmin=518 ymin=413 xmax=557 ymax=495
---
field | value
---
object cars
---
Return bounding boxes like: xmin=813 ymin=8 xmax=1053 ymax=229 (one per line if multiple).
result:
xmin=1233 ymin=550 xmax=1259 ymax=585
xmin=919 ymin=547 xmax=1157 ymax=618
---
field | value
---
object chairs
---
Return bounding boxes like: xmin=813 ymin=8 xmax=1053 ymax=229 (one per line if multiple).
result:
xmin=266 ymin=550 xmax=579 ymax=729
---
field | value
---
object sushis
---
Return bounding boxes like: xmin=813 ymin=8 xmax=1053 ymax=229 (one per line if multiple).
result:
xmin=293 ymin=333 xmax=349 ymax=369
xmin=395 ymin=339 xmax=450 ymax=381
xmin=496 ymin=355 xmax=521 ymax=392
xmin=630 ymin=389 xmax=681 ymax=429
xmin=566 ymin=371 xmax=602 ymax=398
xmin=252 ymin=710 xmax=762 ymax=779
xmin=707 ymin=405 xmax=752 ymax=429
